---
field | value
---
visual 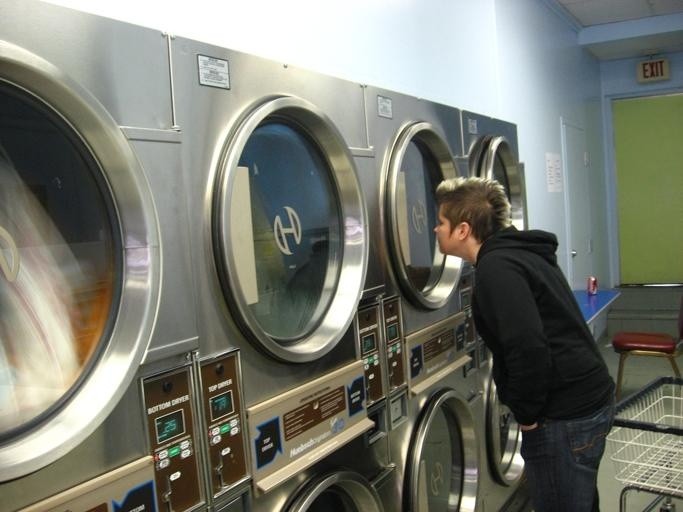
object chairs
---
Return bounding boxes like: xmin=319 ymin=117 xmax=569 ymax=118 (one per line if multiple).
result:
xmin=611 ymin=313 xmax=683 ymax=403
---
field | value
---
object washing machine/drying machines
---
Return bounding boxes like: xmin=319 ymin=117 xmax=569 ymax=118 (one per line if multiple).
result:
xmin=1 ymin=1 xmax=530 ymax=511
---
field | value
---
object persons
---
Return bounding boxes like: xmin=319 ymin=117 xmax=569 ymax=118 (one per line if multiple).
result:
xmin=433 ymin=175 xmax=616 ymax=512
